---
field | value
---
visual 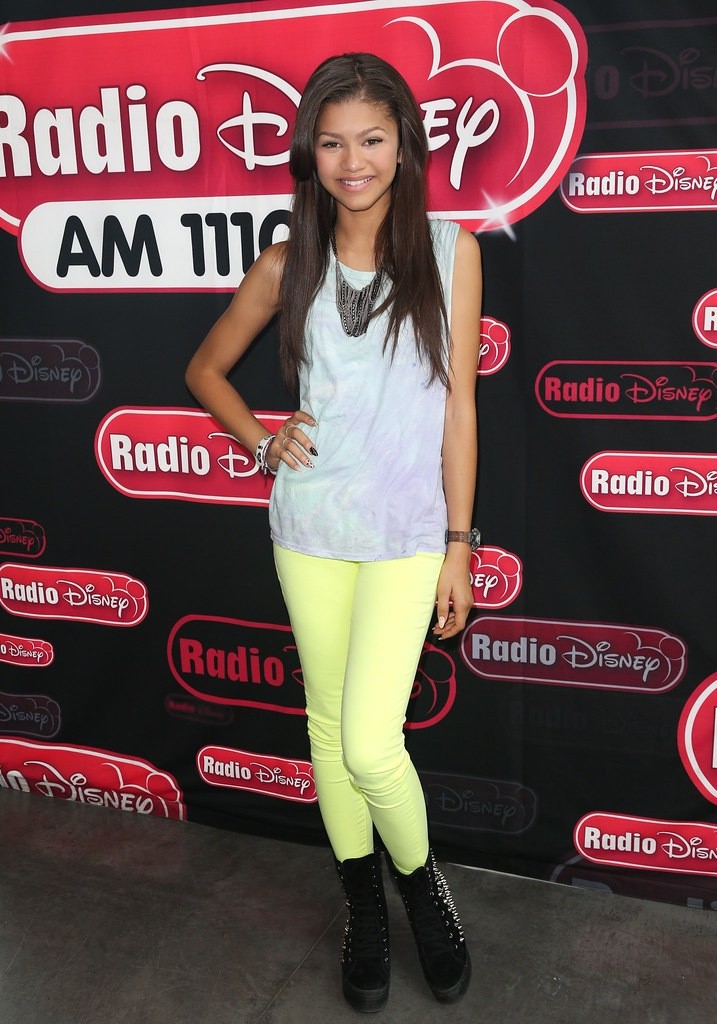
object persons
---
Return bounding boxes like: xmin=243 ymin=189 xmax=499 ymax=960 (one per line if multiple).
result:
xmin=188 ymin=48 xmax=485 ymax=1010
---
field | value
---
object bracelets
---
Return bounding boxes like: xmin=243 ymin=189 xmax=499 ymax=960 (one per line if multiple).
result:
xmin=255 ymin=433 xmax=277 ymax=477
xmin=444 ymin=527 xmax=481 ymax=553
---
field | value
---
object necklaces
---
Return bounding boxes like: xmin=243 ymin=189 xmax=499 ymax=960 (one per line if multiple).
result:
xmin=331 ymin=225 xmax=387 ymax=337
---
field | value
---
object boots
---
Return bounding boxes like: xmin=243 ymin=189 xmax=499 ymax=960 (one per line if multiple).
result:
xmin=332 ymin=839 xmax=392 ymax=1014
xmin=384 ymin=843 xmax=471 ymax=1006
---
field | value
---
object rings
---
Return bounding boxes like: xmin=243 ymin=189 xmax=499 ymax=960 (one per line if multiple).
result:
xmin=282 ymin=437 xmax=290 ymax=448
xmin=285 ymin=425 xmax=293 ymax=436
